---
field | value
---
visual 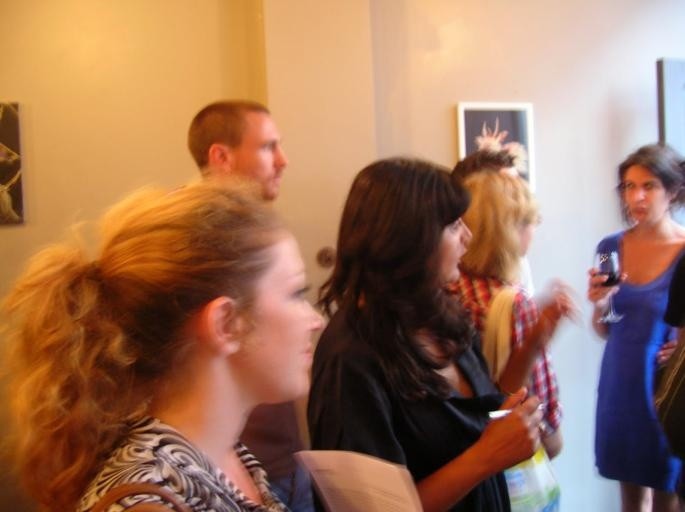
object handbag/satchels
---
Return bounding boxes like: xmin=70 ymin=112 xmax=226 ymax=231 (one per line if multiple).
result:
xmin=486 ymin=406 xmax=567 ymax=512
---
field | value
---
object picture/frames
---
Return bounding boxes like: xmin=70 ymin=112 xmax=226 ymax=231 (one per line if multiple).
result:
xmin=458 ymin=101 xmax=535 ymax=198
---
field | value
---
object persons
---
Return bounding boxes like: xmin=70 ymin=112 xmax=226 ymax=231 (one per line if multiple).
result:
xmin=446 ymin=167 xmax=577 ymax=465
xmin=307 ymin=156 xmax=579 ymax=512
xmin=656 ymin=235 xmax=685 ymax=496
xmin=0 ymin=143 xmax=21 ymax=223
xmin=0 ymin=175 xmax=340 ymax=512
xmin=187 ymin=99 xmax=331 ymax=512
xmin=586 ymin=142 xmax=685 ymax=511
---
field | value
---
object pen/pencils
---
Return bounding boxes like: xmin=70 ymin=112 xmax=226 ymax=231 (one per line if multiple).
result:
xmin=487 ymin=403 xmax=545 ymax=420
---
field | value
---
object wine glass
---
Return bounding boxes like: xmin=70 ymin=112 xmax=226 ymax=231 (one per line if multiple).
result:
xmin=593 ymin=250 xmax=624 ymax=324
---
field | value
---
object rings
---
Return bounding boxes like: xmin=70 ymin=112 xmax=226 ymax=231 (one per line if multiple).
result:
xmin=538 ymin=421 xmax=546 ymax=435
